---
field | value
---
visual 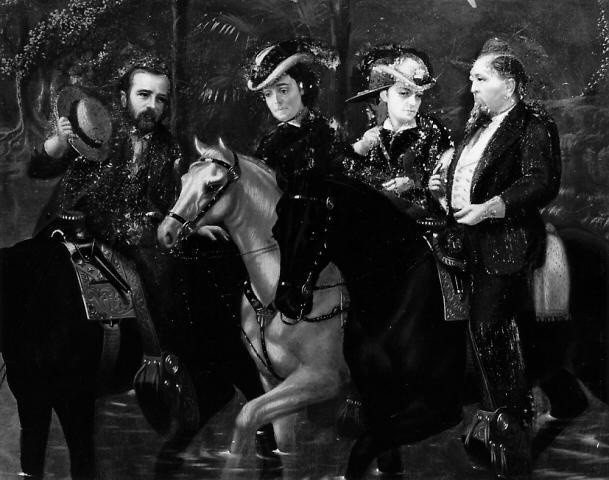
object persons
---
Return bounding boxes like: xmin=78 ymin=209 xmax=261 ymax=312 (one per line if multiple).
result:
xmin=327 ymin=46 xmax=456 ymax=207
xmin=244 ymin=34 xmax=359 ymax=181
xmin=427 ymin=51 xmax=562 ymax=479
xmin=29 ymin=53 xmax=191 ymax=250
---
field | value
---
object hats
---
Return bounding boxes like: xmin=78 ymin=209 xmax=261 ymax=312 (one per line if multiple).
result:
xmin=55 ymin=87 xmax=113 ymax=163
xmin=247 ymin=45 xmax=316 ymax=91
xmin=346 ymin=54 xmax=436 ymax=104
